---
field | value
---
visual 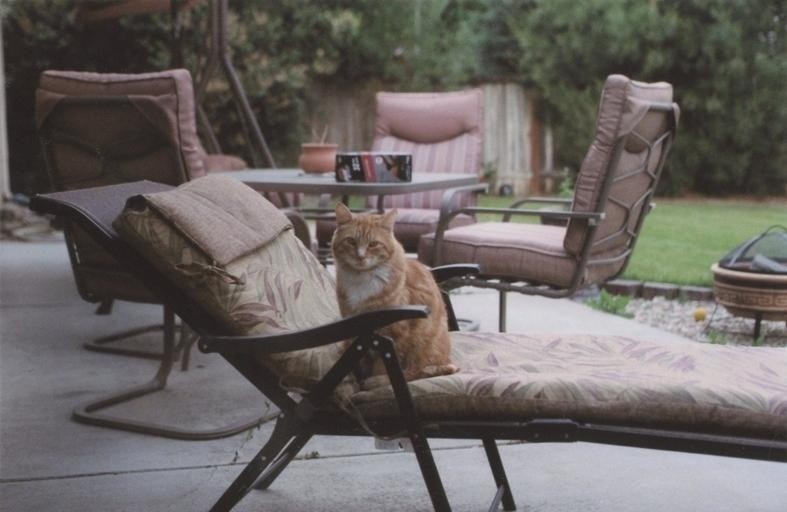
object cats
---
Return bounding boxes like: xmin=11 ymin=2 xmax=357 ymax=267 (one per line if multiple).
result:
xmin=326 ymin=202 xmax=461 ymax=388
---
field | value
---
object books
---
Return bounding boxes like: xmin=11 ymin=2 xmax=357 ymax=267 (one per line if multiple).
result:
xmin=334 ymin=152 xmax=412 ymax=183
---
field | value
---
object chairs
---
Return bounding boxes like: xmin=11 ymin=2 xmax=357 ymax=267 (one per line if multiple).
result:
xmin=315 ymin=84 xmax=489 ymax=271
xmin=31 ymin=66 xmax=259 ymax=364
xmin=415 ymin=73 xmax=682 ymax=330
xmin=25 ymin=168 xmax=787 ymax=512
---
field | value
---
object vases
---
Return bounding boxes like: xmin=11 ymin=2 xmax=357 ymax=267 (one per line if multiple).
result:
xmin=700 ymin=256 xmax=787 ymax=341
